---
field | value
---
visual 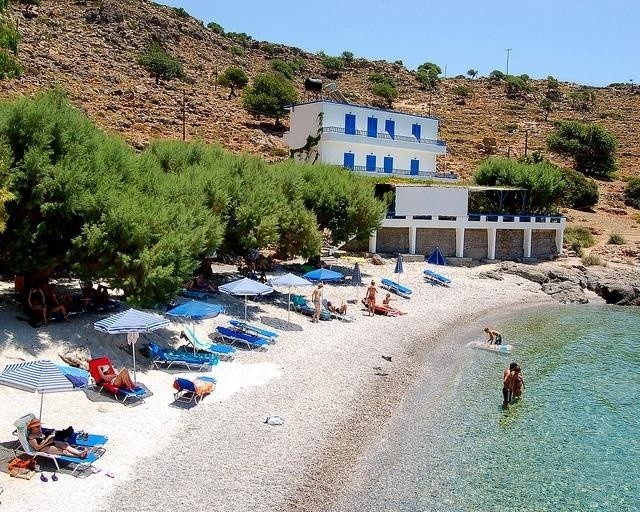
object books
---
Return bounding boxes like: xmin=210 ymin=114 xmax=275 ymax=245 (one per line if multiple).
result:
xmin=44 ymin=431 xmax=55 ymax=444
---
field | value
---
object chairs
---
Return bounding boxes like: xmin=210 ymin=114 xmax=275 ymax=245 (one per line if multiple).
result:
xmin=424 ymin=270 xmax=451 ymax=287
xmin=382 ymin=279 xmax=412 ymax=296
xmin=11 ymin=412 xmax=109 ymax=478
xmin=179 ymin=288 xmax=219 ymax=299
xmin=362 ymin=297 xmax=400 ymax=317
xmin=229 ymin=320 xmax=279 ymax=344
xmin=293 ymin=303 xmax=331 ymax=321
xmin=321 ymin=299 xmax=355 ymax=322
xmin=174 ymin=376 xmax=217 ymax=405
xmin=215 ymin=326 xmax=271 ymax=351
xmin=144 ymin=342 xmax=216 ymax=372
xmin=81 ymin=284 xmax=120 ymax=314
xmin=89 ymin=357 xmax=146 ymax=403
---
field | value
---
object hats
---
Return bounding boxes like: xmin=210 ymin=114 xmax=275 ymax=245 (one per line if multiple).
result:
xmin=27 ymin=419 xmax=40 ymax=429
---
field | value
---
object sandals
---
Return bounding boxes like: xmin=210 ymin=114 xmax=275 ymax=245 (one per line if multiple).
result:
xmin=41 ymin=475 xmax=48 ymax=482
xmin=52 ymin=475 xmax=58 ymax=480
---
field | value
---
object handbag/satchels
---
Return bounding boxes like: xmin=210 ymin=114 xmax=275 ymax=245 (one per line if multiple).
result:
xmin=8 ymin=454 xmax=36 ymax=480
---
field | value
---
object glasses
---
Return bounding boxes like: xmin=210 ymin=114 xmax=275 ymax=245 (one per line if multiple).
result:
xmin=34 ymin=426 xmax=39 ymax=428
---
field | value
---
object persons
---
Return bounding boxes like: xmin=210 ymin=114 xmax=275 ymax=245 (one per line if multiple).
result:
xmin=327 ymin=300 xmax=347 ymax=315
xmin=182 ymin=227 xmax=332 ymax=292
xmin=28 ymin=418 xmax=88 ymax=458
xmin=513 ymin=366 xmax=525 ymax=400
xmin=25 ymin=280 xmax=109 ymax=326
xmin=311 ymin=283 xmax=324 ymax=323
xmin=484 ymin=328 xmax=502 ymax=345
xmin=502 ymin=362 xmax=518 ymax=408
xmin=97 ymin=365 xmax=136 ymax=392
xmin=383 ymin=294 xmax=398 ymax=312
xmin=365 ymin=281 xmax=379 ymax=316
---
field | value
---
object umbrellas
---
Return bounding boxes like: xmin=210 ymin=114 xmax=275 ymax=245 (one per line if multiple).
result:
xmin=93 ymin=308 xmax=171 ymax=384
xmin=271 ymin=273 xmax=312 ymax=327
xmin=395 ymin=253 xmax=404 ymax=284
xmin=219 ymin=277 xmax=274 ymax=330
xmin=166 ymin=298 xmax=224 ymax=355
xmin=353 ymin=261 xmax=362 ymax=297
xmin=0 ymin=358 xmax=82 ymax=430
xmin=304 ymin=268 xmax=343 ymax=310
xmin=428 ymin=246 xmax=446 ymax=272
